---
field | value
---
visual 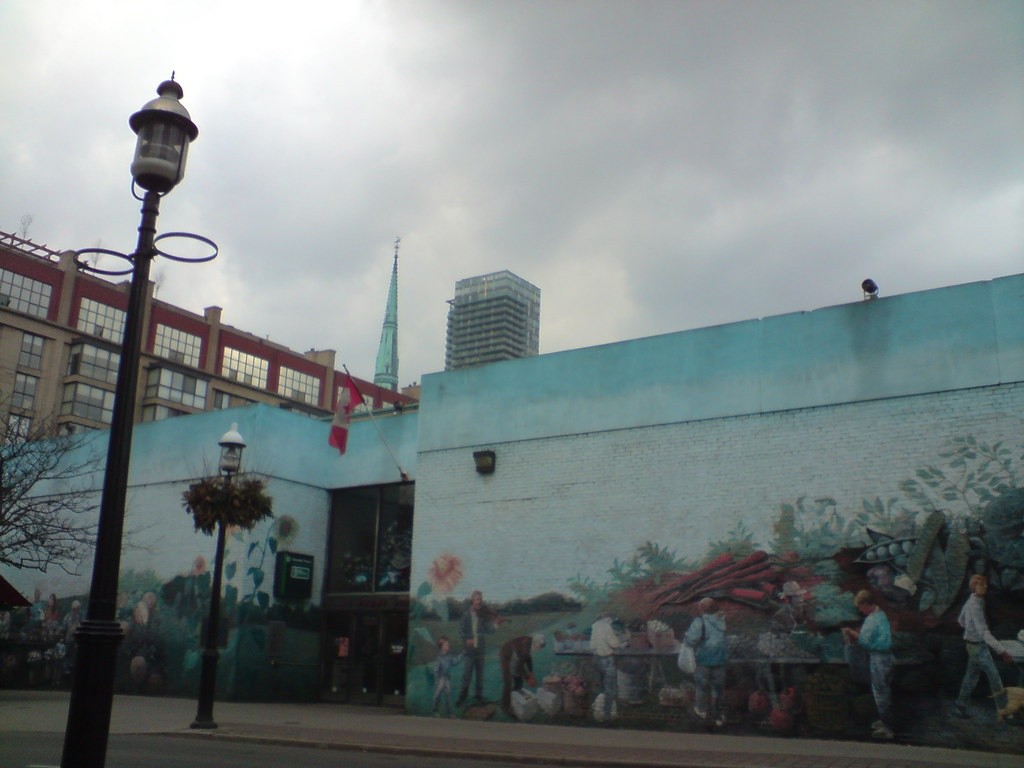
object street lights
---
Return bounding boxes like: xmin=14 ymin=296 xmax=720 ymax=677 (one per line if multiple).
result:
xmin=190 ymin=422 xmax=247 ymax=728
xmin=60 ymin=71 xmax=219 ymax=768
